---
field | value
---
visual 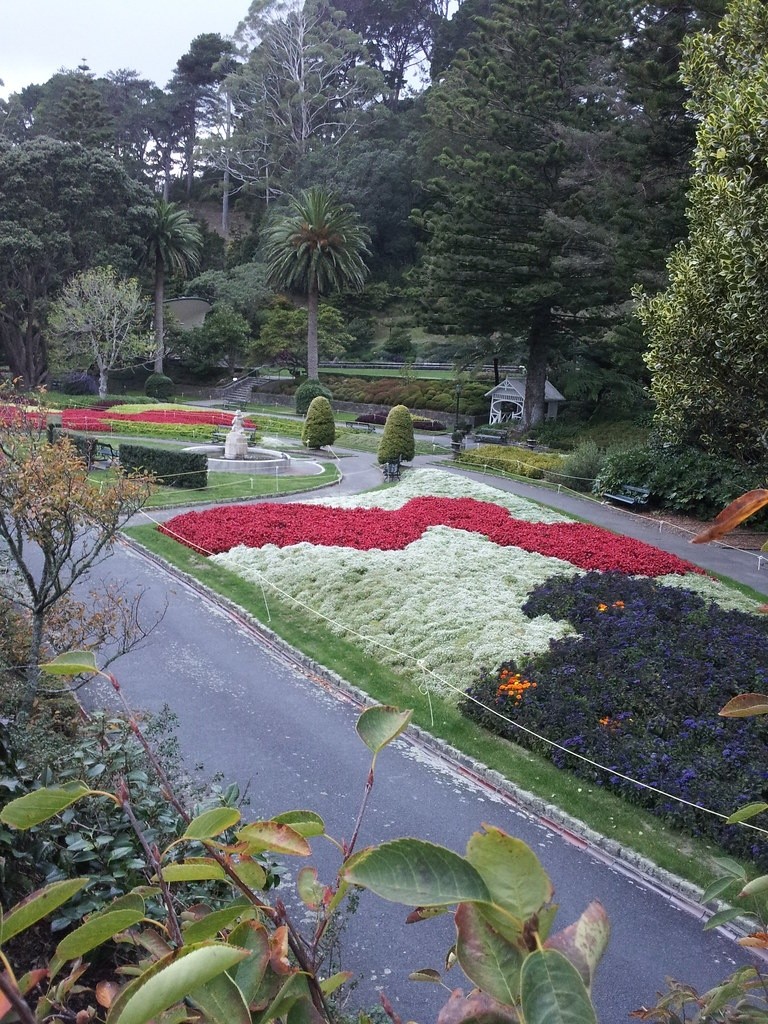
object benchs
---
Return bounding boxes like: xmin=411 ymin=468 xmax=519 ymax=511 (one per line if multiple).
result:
xmin=210 ymin=425 xmax=257 ymax=446
xmin=346 ymin=421 xmax=376 ymax=432
xmin=475 ymin=428 xmax=509 ymax=445
xmin=85 ymin=438 xmax=120 ymax=472
xmin=382 ymin=454 xmax=402 ymax=482
xmin=603 ymin=485 xmax=650 ymax=514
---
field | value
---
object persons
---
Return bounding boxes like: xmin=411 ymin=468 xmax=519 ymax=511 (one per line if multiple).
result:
xmin=231 ymin=409 xmax=246 ymax=434
